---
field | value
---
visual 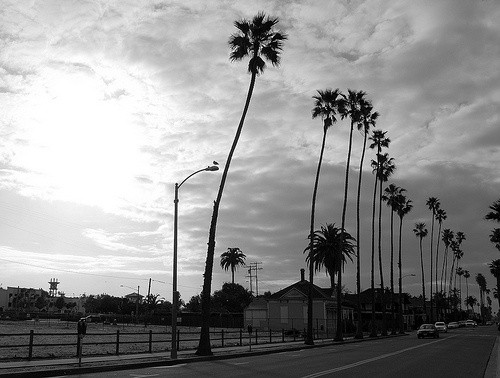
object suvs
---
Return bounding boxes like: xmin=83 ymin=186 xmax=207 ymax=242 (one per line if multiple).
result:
xmin=435 ymin=322 xmax=447 ymax=333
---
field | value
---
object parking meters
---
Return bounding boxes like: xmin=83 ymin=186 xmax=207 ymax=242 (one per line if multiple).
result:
xmin=321 ymin=325 xmax=324 ymax=343
xmin=248 ymin=324 xmax=252 ymax=352
xmin=77 ymin=318 xmax=86 ymax=367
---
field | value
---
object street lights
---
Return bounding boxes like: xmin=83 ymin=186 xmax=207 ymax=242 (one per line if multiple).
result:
xmin=399 ymin=274 xmax=415 ymax=335
xmin=171 ymin=161 xmax=219 ymax=359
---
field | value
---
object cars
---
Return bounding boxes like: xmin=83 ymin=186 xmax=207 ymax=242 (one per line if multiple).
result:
xmin=486 ymin=321 xmax=492 ymax=326
xmin=417 ymin=323 xmax=440 ymax=339
xmin=447 ymin=319 xmax=477 ymax=328
